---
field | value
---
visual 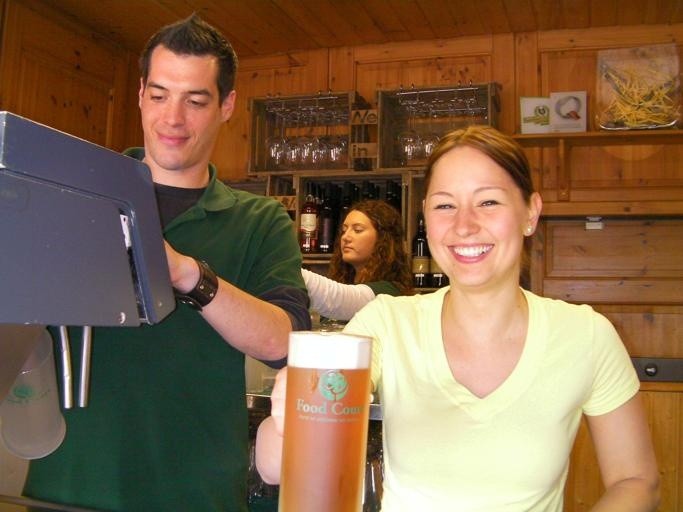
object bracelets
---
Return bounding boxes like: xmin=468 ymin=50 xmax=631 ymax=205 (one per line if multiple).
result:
xmin=177 ymin=256 xmax=221 ymax=312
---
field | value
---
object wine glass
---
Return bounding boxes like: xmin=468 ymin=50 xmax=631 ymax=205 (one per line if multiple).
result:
xmin=392 ymin=100 xmax=483 ymax=159
xmin=264 ymin=102 xmax=348 ymax=171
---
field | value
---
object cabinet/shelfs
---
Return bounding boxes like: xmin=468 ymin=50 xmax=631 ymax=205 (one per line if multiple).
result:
xmin=564 ymin=388 xmax=682 ymax=511
xmin=247 ymin=80 xmax=499 ymax=301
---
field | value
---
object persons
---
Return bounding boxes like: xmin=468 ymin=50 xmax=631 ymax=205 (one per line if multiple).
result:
xmin=253 ymin=121 xmax=663 ymax=512
xmin=17 ymin=10 xmax=309 ymax=512
xmin=297 ymin=198 xmax=418 ymax=324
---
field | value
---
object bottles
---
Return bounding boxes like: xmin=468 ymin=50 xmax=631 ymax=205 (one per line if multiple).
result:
xmin=410 ymin=206 xmax=449 ymax=290
xmin=298 ymin=178 xmax=401 ymax=256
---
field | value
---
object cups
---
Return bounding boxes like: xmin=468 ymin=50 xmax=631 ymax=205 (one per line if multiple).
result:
xmin=273 ymin=326 xmax=373 ymax=508
